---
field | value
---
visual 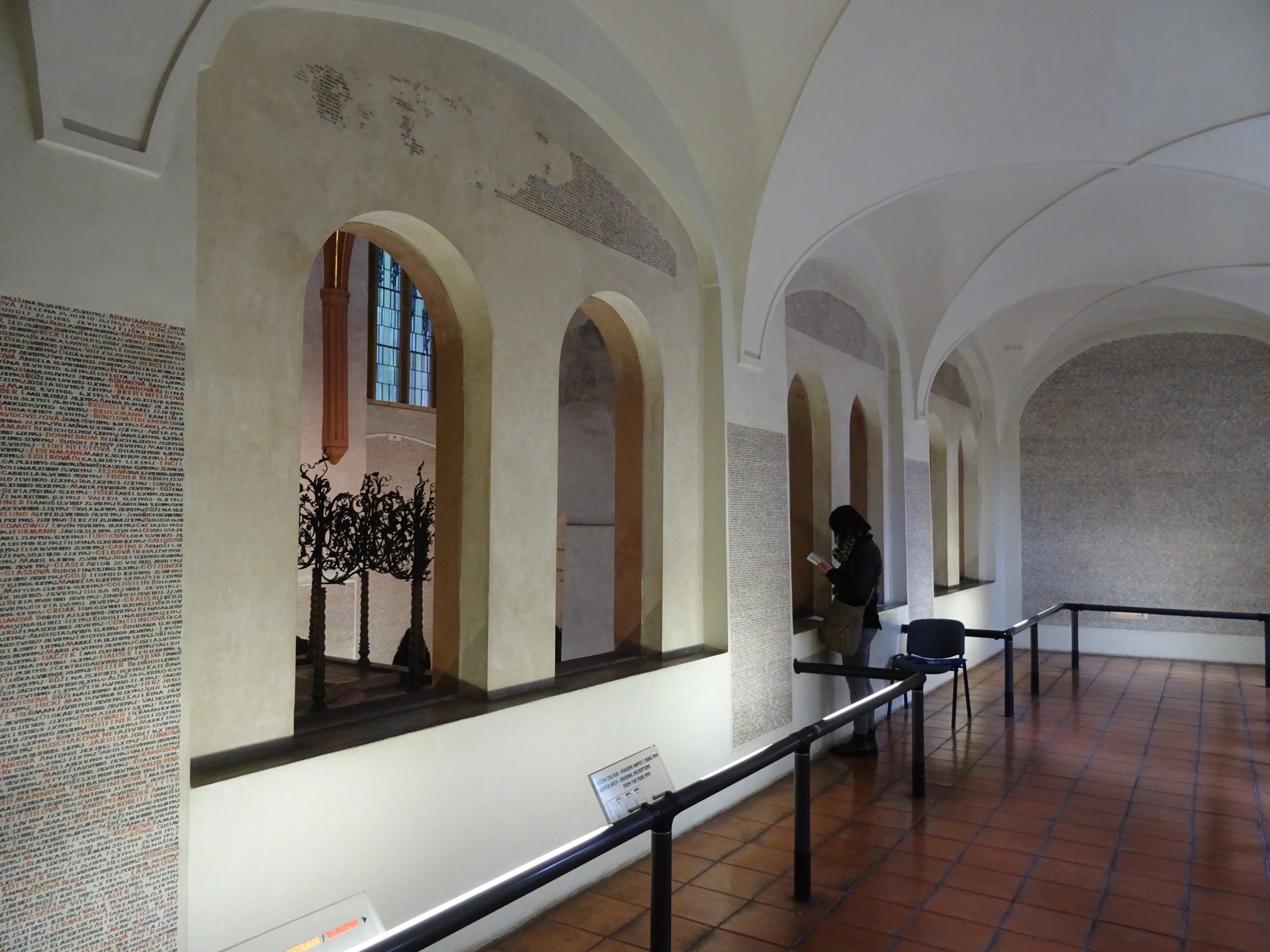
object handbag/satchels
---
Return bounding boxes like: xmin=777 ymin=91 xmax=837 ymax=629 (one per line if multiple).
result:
xmin=818 ymin=599 xmax=866 ymax=655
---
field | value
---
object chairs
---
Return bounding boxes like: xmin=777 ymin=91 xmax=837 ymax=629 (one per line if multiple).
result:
xmin=887 ymin=618 xmax=974 ymax=733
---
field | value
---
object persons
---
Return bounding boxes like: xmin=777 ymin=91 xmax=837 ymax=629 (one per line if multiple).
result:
xmin=818 ymin=504 xmax=881 ymax=759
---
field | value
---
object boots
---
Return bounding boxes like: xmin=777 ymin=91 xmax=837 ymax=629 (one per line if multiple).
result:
xmin=838 ymin=729 xmax=877 ymax=756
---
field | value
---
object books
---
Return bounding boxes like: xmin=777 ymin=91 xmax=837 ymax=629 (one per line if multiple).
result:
xmin=806 ymin=552 xmax=832 ymax=571
